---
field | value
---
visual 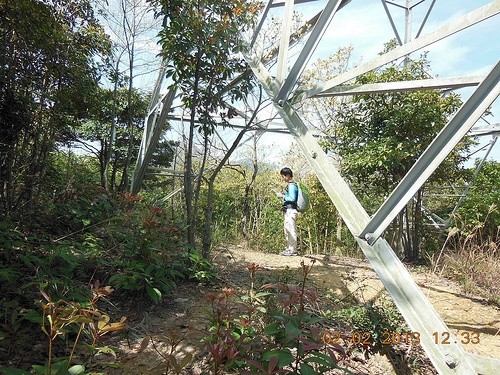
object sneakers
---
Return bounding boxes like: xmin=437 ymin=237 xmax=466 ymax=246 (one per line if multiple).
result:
xmin=281 ymin=250 xmax=297 ymax=255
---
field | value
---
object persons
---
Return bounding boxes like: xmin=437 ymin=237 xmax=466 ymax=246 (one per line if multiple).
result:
xmin=274 ymin=167 xmax=307 ymax=256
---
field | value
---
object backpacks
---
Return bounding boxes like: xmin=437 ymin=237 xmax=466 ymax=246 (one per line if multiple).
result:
xmin=285 ymin=182 xmax=310 ymax=212
